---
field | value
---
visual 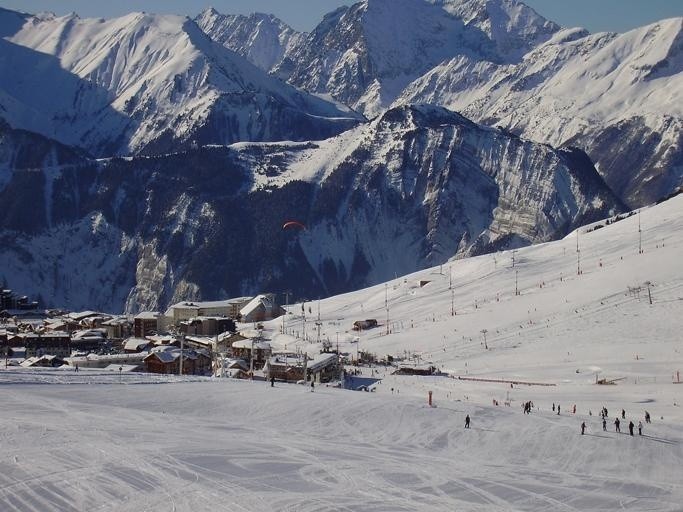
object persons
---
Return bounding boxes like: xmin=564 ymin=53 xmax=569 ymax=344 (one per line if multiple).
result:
xmin=581 ymin=422 xmax=586 ymax=435
xmin=74 ymin=362 xmax=78 ymax=372
xmin=326 ymin=367 xmax=394 ymax=394
xmin=552 ymin=402 xmax=555 ymax=411
xmin=572 ymin=405 xmax=576 ymax=413
xmin=628 ymin=421 xmax=634 ymax=436
xmin=614 ymin=417 xmax=621 ymax=432
xmin=621 ymin=409 xmax=626 ymax=419
xmin=311 ymin=381 xmax=314 ymax=392
xmin=602 ymin=419 xmax=607 ymax=430
xmin=522 ymin=400 xmax=533 ymax=415
xmin=637 ymin=421 xmax=643 ymax=435
xmin=464 ymin=414 xmax=471 ymax=428
xmin=588 ymin=406 xmax=608 ymax=419
xmin=644 ymin=410 xmax=652 ymax=423
xmin=557 ymin=404 xmax=560 ymax=415
xmin=270 ymin=376 xmax=276 ymax=387
